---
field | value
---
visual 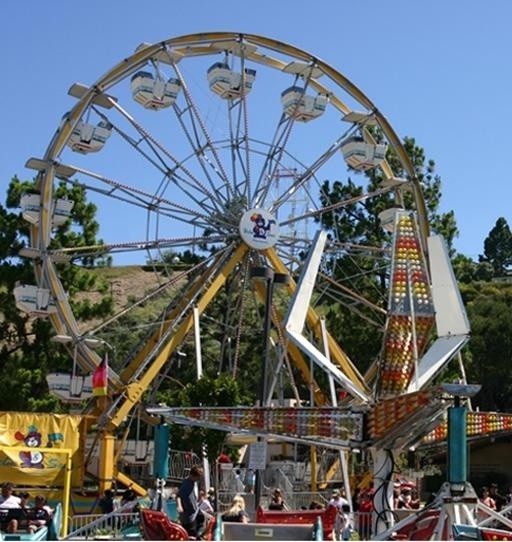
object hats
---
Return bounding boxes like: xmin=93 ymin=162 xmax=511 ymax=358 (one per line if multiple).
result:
xmin=331 ymin=488 xmax=341 ymax=497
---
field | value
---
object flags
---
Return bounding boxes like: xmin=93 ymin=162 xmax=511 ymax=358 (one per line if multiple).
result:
xmin=92 ymin=351 xmax=108 ymax=397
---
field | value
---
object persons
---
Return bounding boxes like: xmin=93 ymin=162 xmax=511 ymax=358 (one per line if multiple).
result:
xmin=198 ymin=487 xmax=250 ymax=523
xmin=175 ymin=466 xmax=205 ymax=538
xmin=478 ymin=483 xmax=511 ymax=526
xmin=393 ymin=487 xmax=420 ymax=509
xmin=243 ymin=468 xmax=255 ymax=492
xmin=98 ymin=490 xmax=139 ymax=530
xmin=0 ymin=479 xmax=53 ymax=534
xmin=271 ymin=488 xmax=283 ymax=505
xmin=300 ymin=489 xmax=350 ymax=541
xmin=352 ymin=479 xmax=374 ymax=541
